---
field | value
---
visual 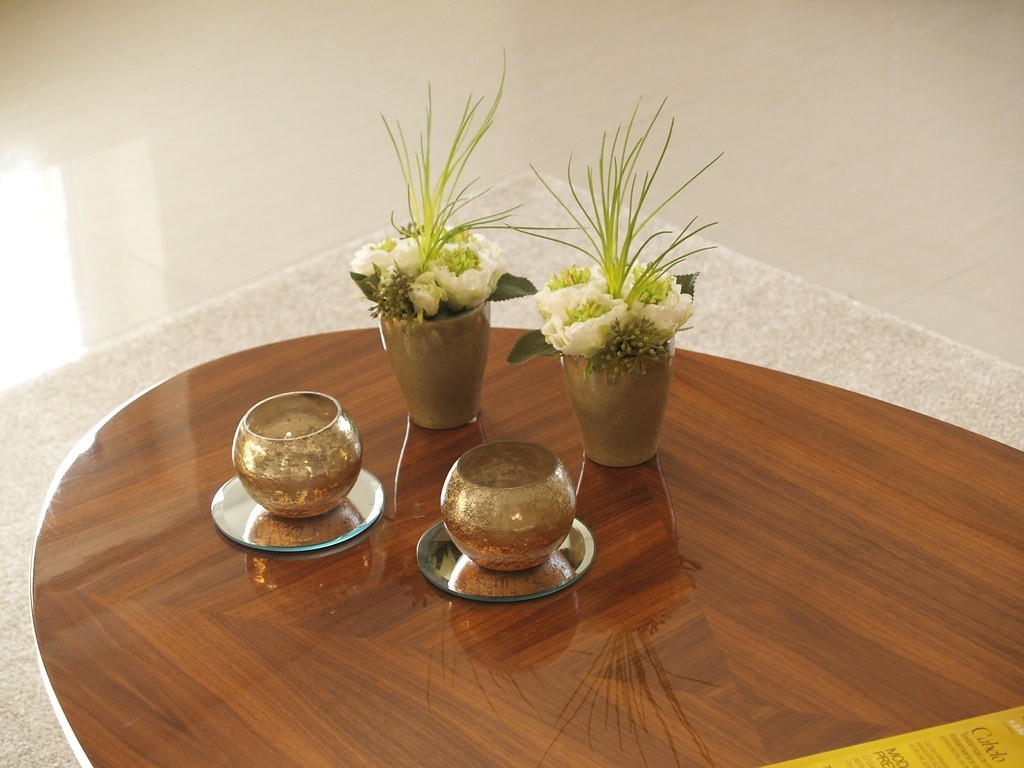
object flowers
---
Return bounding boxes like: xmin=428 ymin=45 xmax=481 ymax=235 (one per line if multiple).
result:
xmin=350 ymin=49 xmax=537 ymax=328
xmin=506 ymin=90 xmax=714 ymax=380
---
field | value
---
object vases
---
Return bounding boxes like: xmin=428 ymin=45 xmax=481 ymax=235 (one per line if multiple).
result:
xmin=377 ymin=301 xmax=491 ymax=429
xmin=559 ymin=340 xmax=674 ymax=467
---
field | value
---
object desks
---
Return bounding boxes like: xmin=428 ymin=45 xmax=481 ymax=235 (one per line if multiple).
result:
xmin=31 ymin=324 xmax=1024 ymax=767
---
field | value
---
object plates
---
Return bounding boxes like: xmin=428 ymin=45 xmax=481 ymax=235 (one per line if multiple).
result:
xmin=416 ymin=515 xmax=596 ymax=604
xmin=211 ymin=469 xmax=385 ymax=554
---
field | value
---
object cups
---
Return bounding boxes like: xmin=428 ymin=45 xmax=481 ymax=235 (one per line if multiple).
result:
xmin=439 ymin=441 xmax=577 ymax=572
xmin=232 ymin=391 xmax=364 ymax=520
